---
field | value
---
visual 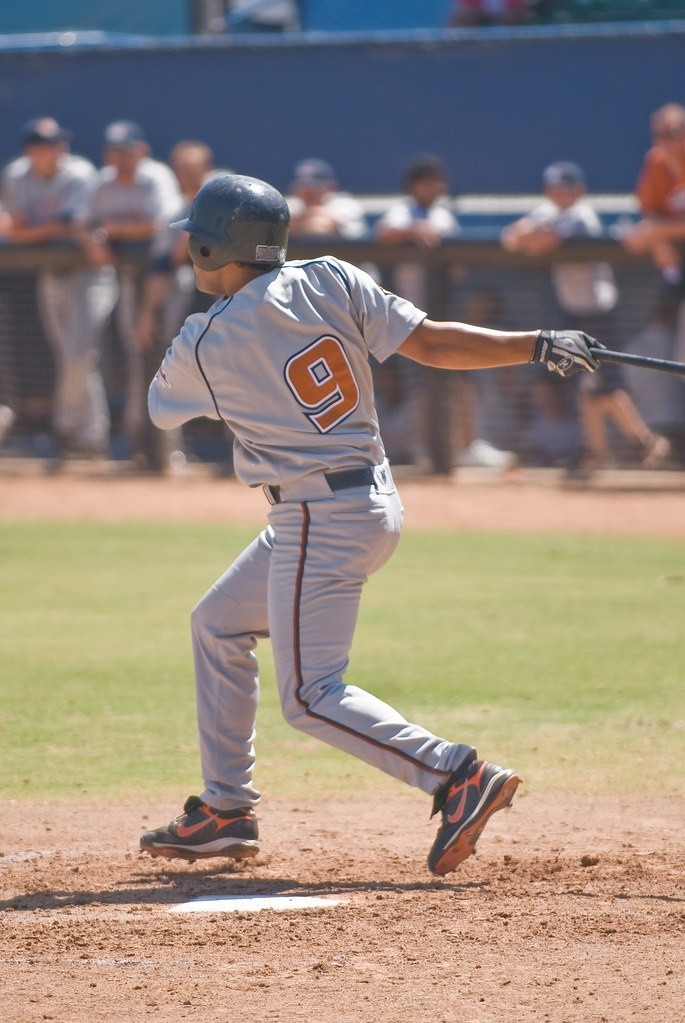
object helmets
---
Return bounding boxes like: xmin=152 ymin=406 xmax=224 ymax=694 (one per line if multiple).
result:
xmin=169 ymin=175 xmax=290 ymax=271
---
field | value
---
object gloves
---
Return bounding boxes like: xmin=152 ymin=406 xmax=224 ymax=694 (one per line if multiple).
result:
xmin=529 ymin=329 xmax=607 ymax=378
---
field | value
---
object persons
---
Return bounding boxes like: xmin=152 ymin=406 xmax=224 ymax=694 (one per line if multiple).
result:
xmin=0 ymin=102 xmax=684 ymax=472
xmin=136 ymin=176 xmax=609 ymax=876
xmin=199 ymin=0 xmax=301 ymax=37
xmin=450 ymin=0 xmax=541 ymax=29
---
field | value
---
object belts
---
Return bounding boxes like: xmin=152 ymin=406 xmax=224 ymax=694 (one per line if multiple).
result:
xmin=269 ymin=468 xmax=374 ymax=502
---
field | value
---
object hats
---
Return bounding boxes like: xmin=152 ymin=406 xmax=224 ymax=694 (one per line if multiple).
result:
xmin=294 ymin=161 xmax=334 ymax=183
xmin=20 ymin=117 xmax=74 ymax=144
xmin=104 ymin=120 xmax=145 ymax=145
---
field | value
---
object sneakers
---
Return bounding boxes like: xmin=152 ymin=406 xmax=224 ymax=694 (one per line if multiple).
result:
xmin=140 ymin=795 xmax=259 ymax=864
xmin=427 ymin=759 xmax=523 ymax=876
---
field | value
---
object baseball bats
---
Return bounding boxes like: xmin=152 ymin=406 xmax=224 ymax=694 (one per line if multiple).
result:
xmin=589 ymin=345 xmax=685 ymax=377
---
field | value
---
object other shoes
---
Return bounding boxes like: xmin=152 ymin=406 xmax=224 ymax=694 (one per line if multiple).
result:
xmin=460 ymin=438 xmax=517 ymax=472
